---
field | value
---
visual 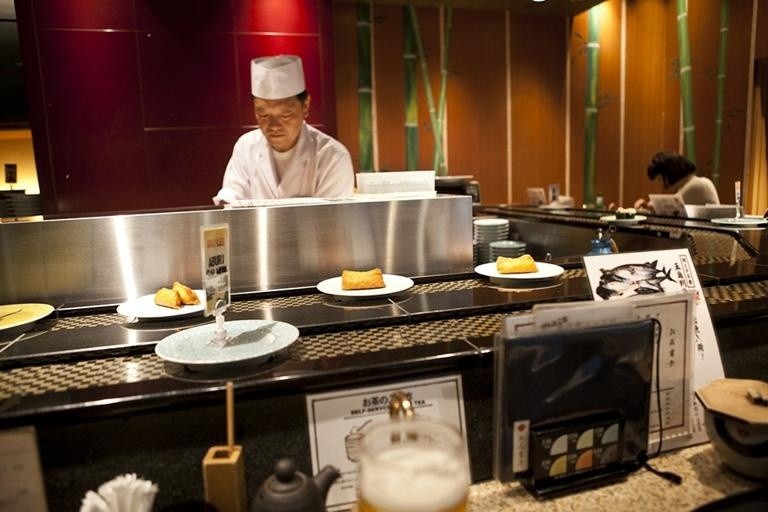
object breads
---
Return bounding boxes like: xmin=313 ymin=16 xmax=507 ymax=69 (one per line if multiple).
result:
xmin=496 ymin=254 xmax=538 ymax=274
xmin=342 ymin=268 xmax=384 ymax=290
xmin=155 ymin=287 xmax=181 ymax=309
xmin=173 ymin=281 xmax=200 ymax=305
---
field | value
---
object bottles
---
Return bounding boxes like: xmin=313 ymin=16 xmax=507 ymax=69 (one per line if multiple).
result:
xmin=595 ymin=190 xmax=605 ymax=208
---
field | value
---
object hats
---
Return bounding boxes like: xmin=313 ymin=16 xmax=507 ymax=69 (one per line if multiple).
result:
xmin=250 ymin=55 xmax=305 ymax=101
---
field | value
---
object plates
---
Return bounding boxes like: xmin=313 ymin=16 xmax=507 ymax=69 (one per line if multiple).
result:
xmin=710 ymin=218 xmax=765 ymax=226
xmin=150 ymin=318 xmax=300 ymax=368
xmin=117 ymin=290 xmax=212 ymax=319
xmin=311 ymin=273 xmax=417 ymax=299
xmin=472 ymin=219 xmax=528 ymax=267
xmin=472 ymin=262 xmax=568 ymax=288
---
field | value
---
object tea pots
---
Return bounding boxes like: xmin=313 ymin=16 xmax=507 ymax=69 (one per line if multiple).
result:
xmin=253 ymin=457 xmax=340 ymax=512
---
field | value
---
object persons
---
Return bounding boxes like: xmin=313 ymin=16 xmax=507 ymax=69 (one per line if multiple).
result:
xmin=645 ymin=150 xmax=725 ymax=204
xmin=212 ymin=53 xmax=357 ymax=203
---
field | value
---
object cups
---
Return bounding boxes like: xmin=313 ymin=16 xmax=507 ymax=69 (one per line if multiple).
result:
xmin=353 ymin=415 xmax=474 ymax=510
